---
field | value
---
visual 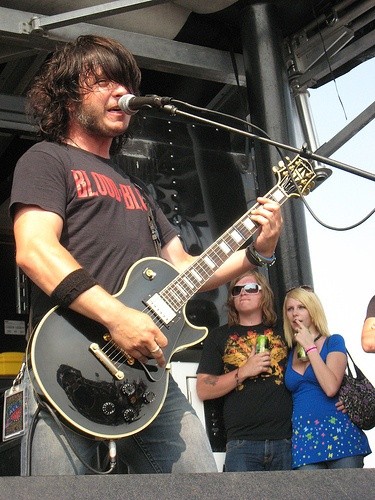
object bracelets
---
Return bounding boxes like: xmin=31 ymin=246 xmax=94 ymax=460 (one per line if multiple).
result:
xmin=50 ymin=269 xmax=100 ymax=307
xmin=235 ymin=368 xmax=244 ymax=392
xmin=245 ymin=242 xmax=276 ymax=267
xmin=307 ymin=347 xmax=317 ymax=352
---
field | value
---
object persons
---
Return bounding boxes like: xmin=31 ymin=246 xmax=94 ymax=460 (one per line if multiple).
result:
xmin=362 ymin=294 xmax=375 ymax=353
xmin=10 ymin=33 xmax=284 ymax=475
xmin=195 ymin=270 xmax=347 ymax=472
xmin=284 ymin=284 xmax=372 ymax=472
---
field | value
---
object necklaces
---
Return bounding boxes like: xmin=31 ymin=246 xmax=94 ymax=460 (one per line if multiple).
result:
xmin=314 ymin=334 xmax=322 ymax=342
xmin=66 ymin=136 xmax=80 ymax=148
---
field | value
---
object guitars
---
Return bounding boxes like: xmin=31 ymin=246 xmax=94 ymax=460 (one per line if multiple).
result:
xmin=25 ymin=153 xmax=317 ymax=443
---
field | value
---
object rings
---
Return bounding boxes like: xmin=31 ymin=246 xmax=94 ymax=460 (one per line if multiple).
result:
xmin=294 ymin=333 xmax=297 ymax=336
xmin=151 ymin=346 xmax=159 ymax=353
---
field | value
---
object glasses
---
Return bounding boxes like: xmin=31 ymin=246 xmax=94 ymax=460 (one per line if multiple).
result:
xmin=285 ymin=285 xmax=314 ymax=295
xmin=231 ymin=283 xmax=262 ymax=297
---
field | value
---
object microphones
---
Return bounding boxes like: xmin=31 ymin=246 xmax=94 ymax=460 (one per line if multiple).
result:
xmin=117 ymin=94 xmax=172 ymax=116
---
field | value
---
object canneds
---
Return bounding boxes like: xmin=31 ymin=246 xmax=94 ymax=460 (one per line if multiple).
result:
xmin=255 ymin=334 xmax=269 ymax=354
xmin=296 ymin=342 xmax=309 ymax=361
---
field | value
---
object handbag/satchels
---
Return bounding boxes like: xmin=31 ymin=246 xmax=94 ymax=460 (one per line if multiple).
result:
xmin=202 ymin=342 xmax=225 ymax=452
xmin=326 ymin=336 xmax=375 ymax=430
